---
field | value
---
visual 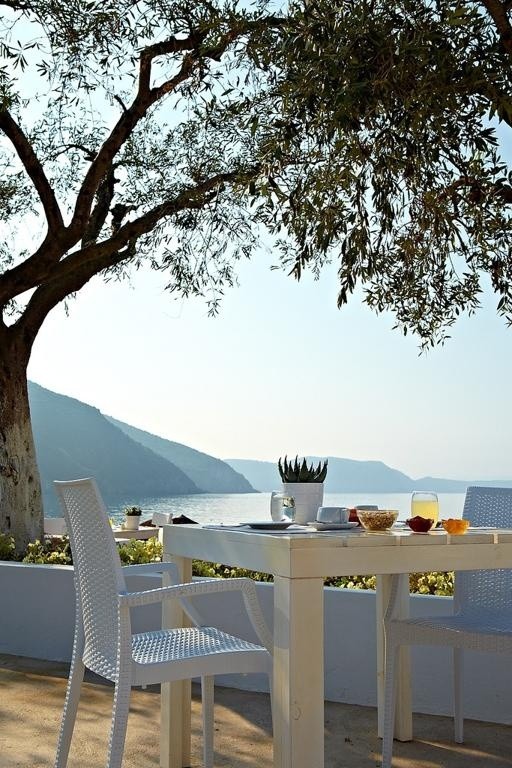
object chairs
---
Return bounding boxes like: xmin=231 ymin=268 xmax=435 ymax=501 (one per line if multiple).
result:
xmin=152 ymin=511 xmax=173 ymax=530
xmin=382 ymin=486 xmax=512 ymax=767
xmin=53 ymin=476 xmax=274 ymax=767
xmin=44 ymin=518 xmax=68 ymax=540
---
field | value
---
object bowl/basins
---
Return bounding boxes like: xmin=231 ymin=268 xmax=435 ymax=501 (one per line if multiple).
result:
xmin=441 ymin=519 xmax=470 ymax=535
xmin=356 ymin=510 xmax=399 ymax=531
xmin=406 ymin=519 xmax=434 ymax=532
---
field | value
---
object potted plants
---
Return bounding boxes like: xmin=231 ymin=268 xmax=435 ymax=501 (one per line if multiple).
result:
xmin=277 ymin=455 xmax=329 ymax=522
xmin=124 ymin=505 xmax=141 ymax=530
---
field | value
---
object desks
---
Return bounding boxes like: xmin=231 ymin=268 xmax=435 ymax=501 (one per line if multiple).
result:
xmin=111 ymin=525 xmax=159 ymax=540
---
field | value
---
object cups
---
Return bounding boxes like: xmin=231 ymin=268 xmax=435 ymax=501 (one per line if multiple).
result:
xmin=270 ymin=491 xmax=296 ymax=527
xmin=316 ymin=507 xmax=350 ymax=524
xmin=411 ymin=491 xmax=439 ymax=531
xmin=355 ymin=505 xmax=377 ymax=511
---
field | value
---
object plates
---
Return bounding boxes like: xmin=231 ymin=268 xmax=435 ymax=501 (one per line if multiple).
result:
xmin=240 ymin=521 xmax=296 ymax=530
xmin=307 ymin=522 xmax=359 ymax=530
